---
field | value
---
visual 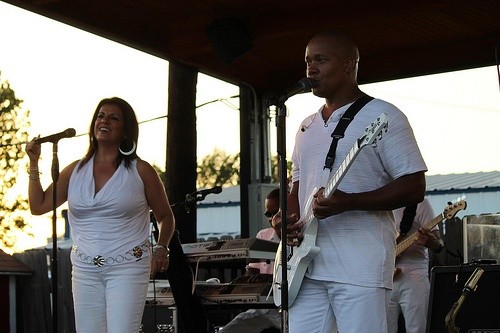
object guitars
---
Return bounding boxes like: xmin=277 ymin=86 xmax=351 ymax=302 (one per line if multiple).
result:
xmin=393 ymin=200 xmax=468 ymax=281
xmin=273 ymin=112 xmax=389 ymax=308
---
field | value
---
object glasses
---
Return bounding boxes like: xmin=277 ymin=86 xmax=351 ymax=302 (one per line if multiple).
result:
xmin=264 ymin=209 xmax=279 ymax=218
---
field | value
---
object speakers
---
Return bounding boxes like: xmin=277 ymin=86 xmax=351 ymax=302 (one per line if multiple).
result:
xmin=425 ymin=264 xmax=500 ymax=333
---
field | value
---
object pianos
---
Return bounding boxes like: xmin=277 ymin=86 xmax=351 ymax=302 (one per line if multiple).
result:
xmin=148 ymin=237 xmax=279 ymax=266
xmin=145 ymin=273 xmax=276 ymax=308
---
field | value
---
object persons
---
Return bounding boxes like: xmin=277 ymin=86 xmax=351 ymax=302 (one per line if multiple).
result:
xmin=25 ymin=97 xmax=176 ymax=333
xmin=213 ymin=189 xmax=289 ymax=333
xmin=271 ymin=27 xmax=428 ymax=333
xmin=388 ymin=197 xmax=444 ymax=333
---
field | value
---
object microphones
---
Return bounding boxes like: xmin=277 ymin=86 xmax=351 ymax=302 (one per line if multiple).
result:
xmin=265 ymin=78 xmax=314 ymax=106
xmin=190 ymin=186 xmax=222 ymax=195
xmin=35 ymin=128 xmax=76 ymax=145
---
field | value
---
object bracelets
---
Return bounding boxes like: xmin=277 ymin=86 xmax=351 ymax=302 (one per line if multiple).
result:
xmin=152 ymin=244 xmax=170 ymax=259
xmin=28 ymin=167 xmax=40 ymax=180
